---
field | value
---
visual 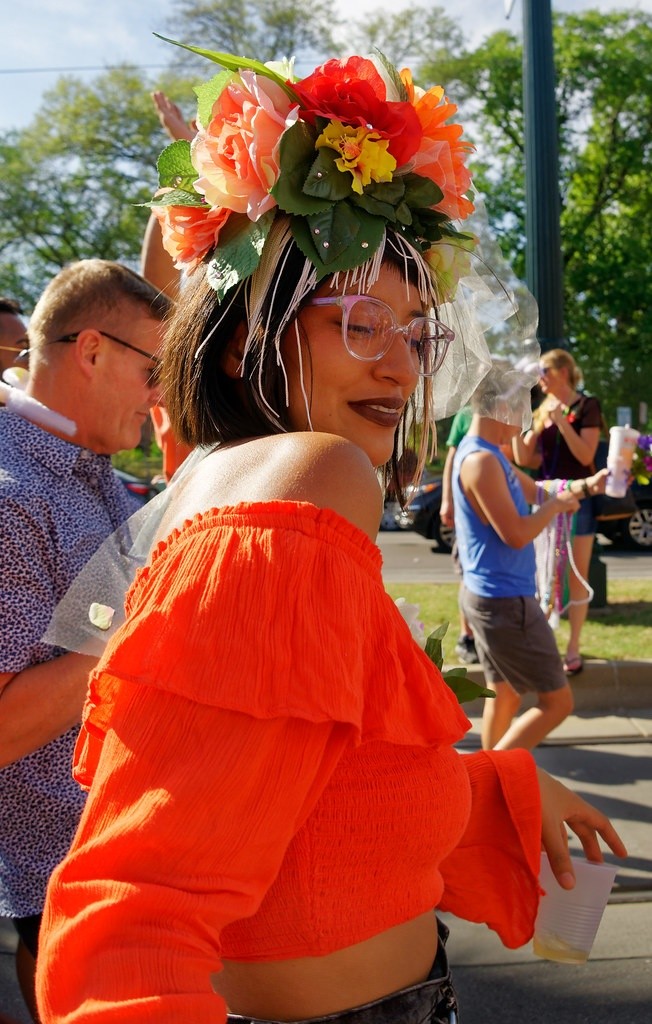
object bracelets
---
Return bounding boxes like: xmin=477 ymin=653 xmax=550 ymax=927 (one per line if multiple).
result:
xmin=581 ymin=478 xmax=591 ymax=499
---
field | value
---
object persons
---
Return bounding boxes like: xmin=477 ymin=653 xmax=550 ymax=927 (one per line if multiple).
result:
xmin=512 ymin=346 xmax=601 ymax=676
xmin=0 ymin=259 xmax=182 ymax=1024
xmin=0 ymin=297 xmax=31 ymax=407
xmin=32 ymin=55 xmax=631 ymax=1024
xmin=439 ymin=355 xmax=636 ymax=751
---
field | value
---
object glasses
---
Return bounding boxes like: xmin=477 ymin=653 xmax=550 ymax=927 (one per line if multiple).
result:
xmin=306 ymin=294 xmax=455 ymax=377
xmin=47 ymin=329 xmax=167 ymax=390
xmin=541 ymin=367 xmax=557 ymax=374
xmin=0 ymin=346 xmax=29 ymax=363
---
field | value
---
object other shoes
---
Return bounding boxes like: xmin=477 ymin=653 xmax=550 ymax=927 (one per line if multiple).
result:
xmin=454 ymin=630 xmax=478 ymax=665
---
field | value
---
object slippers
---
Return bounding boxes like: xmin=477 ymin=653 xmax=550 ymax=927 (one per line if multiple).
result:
xmin=562 ymin=653 xmax=584 ymax=678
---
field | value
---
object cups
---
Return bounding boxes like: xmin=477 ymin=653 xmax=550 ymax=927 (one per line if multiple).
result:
xmin=533 ymin=850 xmax=620 ymax=964
xmin=606 ymin=426 xmax=640 ymax=497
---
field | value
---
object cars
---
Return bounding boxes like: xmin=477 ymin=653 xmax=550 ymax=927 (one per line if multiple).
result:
xmin=394 ymin=437 xmax=652 ymax=554
xmin=111 ymin=466 xmax=150 ymax=504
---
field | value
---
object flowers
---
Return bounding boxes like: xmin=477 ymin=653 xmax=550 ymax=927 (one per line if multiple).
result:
xmin=630 ymin=434 xmax=652 ymax=486
xmin=393 ymin=597 xmax=497 ymax=704
xmin=131 ymin=31 xmax=481 ymax=309
